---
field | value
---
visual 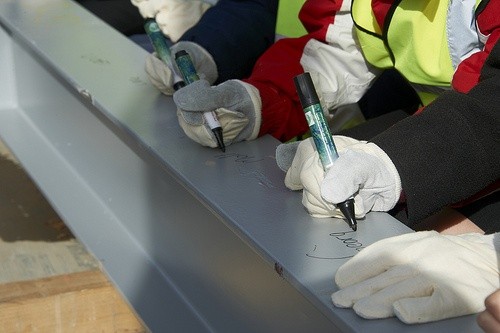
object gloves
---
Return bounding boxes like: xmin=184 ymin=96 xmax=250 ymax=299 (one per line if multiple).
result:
xmin=143 ymin=41 xmax=218 ymax=95
xmin=172 ymin=81 xmax=262 ymax=148
xmin=330 ymin=231 xmax=500 ymax=324
xmin=300 ymin=142 xmax=402 ymax=219
xmin=275 ymin=135 xmax=367 ymax=191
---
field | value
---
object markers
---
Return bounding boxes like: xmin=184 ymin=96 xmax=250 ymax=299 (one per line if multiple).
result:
xmin=142 ymin=17 xmax=186 ymax=93
xmin=175 ymin=50 xmax=227 ymax=155
xmin=294 ymin=71 xmax=358 ymax=233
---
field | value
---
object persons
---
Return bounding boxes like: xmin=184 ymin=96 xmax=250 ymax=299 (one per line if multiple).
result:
xmin=130 ymin=0 xmax=500 ymax=333
